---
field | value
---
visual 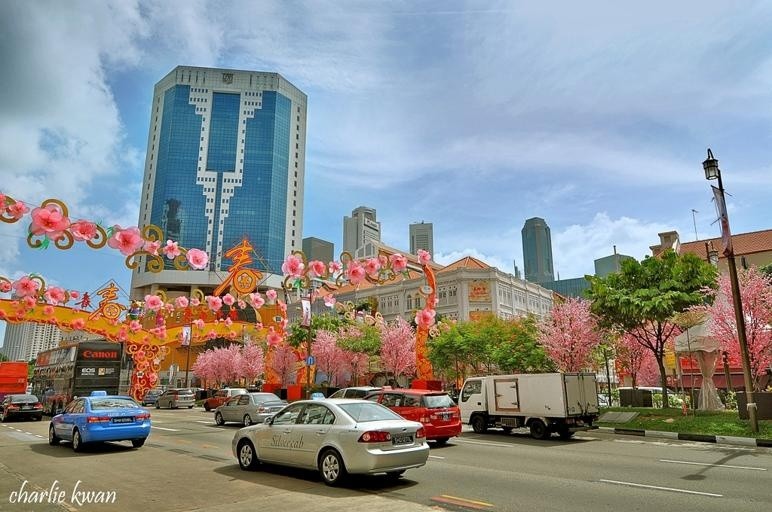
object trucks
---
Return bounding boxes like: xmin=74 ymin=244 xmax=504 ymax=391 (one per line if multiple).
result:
xmin=457 ymin=371 xmax=601 ymax=440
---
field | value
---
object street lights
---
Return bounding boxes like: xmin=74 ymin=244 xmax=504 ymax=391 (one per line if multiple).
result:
xmin=703 ymin=240 xmax=734 ymax=392
xmin=702 ymin=147 xmax=759 ymax=434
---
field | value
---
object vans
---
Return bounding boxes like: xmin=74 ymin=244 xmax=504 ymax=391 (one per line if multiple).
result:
xmin=327 ymin=386 xmax=463 ymax=444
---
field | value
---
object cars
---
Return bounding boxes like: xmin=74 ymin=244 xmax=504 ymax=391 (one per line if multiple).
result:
xmin=47 ymin=390 xmax=152 ymax=453
xmin=231 ymin=397 xmax=430 ymax=487
xmin=203 ymin=388 xmax=250 ymax=412
xmin=0 ymin=393 xmax=44 ymax=422
xmin=214 ymin=392 xmax=289 ymax=427
xmin=142 ymin=387 xmax=196 ymax=409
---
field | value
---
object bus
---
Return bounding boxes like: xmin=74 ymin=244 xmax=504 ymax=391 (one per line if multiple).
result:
xmin=30 ymin=340 xmax=122 ymax=418
